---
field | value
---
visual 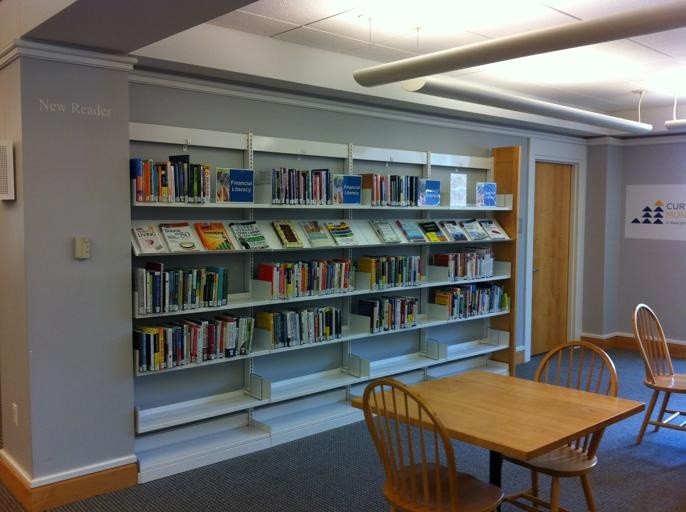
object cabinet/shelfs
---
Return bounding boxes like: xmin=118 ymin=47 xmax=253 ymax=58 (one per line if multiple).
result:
xmin=129 ymin=122 xmax=522 ymax=485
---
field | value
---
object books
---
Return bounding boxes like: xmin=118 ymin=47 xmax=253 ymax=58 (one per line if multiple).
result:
xmin=433 ymin=246 xmax=509 ymax=319
xmin=416 ymin=218 xmax=505 ymax=241
xmin=255 ymin=168 xmax=361 ymax=349
xmin=476 ymin=182 xmax=497 ymax=206
xmin=355 ymin=173 xmax=427 ymax=333
xmin=132 ymin=153 xmax=269 ymax=373
xmin=421 ymin=180 xmax=440 ymax=206
xmin=450 ymin=174 xmax=467 ymax=206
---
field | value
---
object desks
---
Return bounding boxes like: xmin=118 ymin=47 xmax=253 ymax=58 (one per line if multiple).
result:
xmin=351 ymin=370 xmax=645 ymax=511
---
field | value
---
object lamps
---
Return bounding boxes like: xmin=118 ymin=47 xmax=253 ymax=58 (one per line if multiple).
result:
xmin=663 ymin=96 xmax=685 ymax=130
xmin=402 ymin=27 xmax=654 ymax=136
xmin=352 ymin=3 xmax=685 ymax=91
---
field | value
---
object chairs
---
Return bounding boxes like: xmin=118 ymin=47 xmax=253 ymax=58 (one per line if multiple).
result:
xmin=363 ymin=379 xmax=506 ymax=511
xmin=500 ymin=340 xmax=617 ymax=512
xmin=632 ymin=302 xmax=686 ymax=447
